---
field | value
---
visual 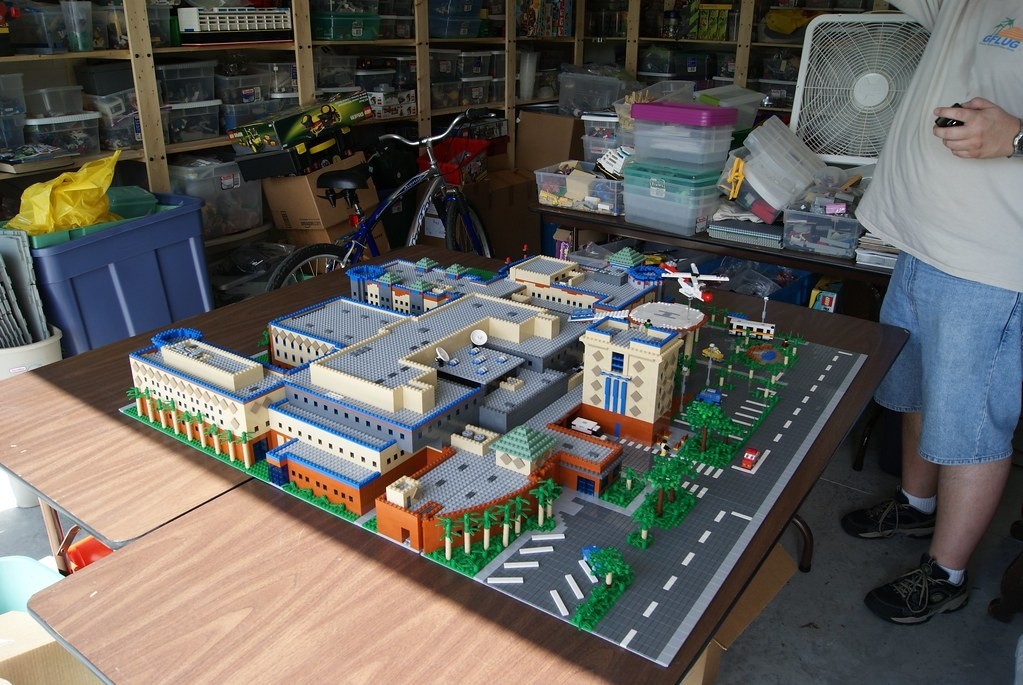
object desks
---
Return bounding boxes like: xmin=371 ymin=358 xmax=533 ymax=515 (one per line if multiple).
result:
xmin=0 ymin=244 xmax=525 ymax=573
xmin=26 ymin=289 xmax=913 ymax=685
xmin=529 ymin=207 xmax=898 ymax=315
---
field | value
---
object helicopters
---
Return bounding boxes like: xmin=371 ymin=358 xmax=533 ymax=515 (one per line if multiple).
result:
xmin=659 ymin=261 xmax=730 ymax=302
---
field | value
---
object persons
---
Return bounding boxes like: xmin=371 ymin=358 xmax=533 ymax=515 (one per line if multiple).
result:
xmin=840 ymin=1 xmax=1023 ymax=623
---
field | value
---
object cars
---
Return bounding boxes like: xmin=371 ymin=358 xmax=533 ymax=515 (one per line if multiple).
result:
xmin=702 ymin=347 xmax=724 ymax=361
xmin=582 ymin=545 xmax=606 ymax=578
xmin=695 ymin=388 xmax=722 ymax=405
xmin=741 ymin=447 xmax=761 ymax=470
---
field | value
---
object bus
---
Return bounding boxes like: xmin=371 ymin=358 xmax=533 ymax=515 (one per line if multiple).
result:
xmin=728 ymin=318 xmax=776 ymax=341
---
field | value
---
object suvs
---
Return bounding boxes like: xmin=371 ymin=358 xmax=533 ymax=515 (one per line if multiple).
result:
xmin=302 ymin=105 xmax=342 ymax=138
xmin=245 ymin=128 xmax=268 ymax=154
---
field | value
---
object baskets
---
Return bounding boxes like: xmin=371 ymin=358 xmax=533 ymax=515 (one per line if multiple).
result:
xmin=416 ymin=136 xmax=491 ymax=187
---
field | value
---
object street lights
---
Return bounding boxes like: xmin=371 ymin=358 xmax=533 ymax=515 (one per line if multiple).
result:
xmin=762 ymin=297 xmax=769 ymax=322
xmin=706 ymin=343 xmax=715 ymax=386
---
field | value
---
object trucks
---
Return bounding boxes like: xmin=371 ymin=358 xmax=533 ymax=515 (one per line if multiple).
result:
xmin=572 ymin=417 xmax=608 ymax=441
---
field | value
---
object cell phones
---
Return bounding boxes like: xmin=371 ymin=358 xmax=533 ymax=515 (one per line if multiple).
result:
xmin=935 ymin=102 xmax=964 ymax=128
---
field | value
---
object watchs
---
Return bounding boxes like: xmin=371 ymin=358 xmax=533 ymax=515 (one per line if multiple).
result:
xmin=1007 ymin=119 xmax=1023 ymax=160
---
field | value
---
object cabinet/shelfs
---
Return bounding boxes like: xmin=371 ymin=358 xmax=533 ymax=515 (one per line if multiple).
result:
xmin=0 ymin=0 xmax=574 ymax=194
xmin=575 ymin=0 xmax=891 ymax=119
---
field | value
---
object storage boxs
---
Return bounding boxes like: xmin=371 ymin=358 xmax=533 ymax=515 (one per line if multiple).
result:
xmin=0 ymin=0 xmax=919 ymax=270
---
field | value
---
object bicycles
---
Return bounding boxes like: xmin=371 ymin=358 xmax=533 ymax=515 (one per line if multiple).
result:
xmin=264 ymin=107 xmax=496 ymax=293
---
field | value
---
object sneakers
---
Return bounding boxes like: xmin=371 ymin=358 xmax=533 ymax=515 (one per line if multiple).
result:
xmin=864 ymin=552 xmax=969 ymax=626
xmin=840 ymin=484 xmax=938 ymax=540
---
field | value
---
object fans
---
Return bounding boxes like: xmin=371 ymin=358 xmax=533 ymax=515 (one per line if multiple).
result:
xmin=793 ymin=14 xmax=933 ymax=162
